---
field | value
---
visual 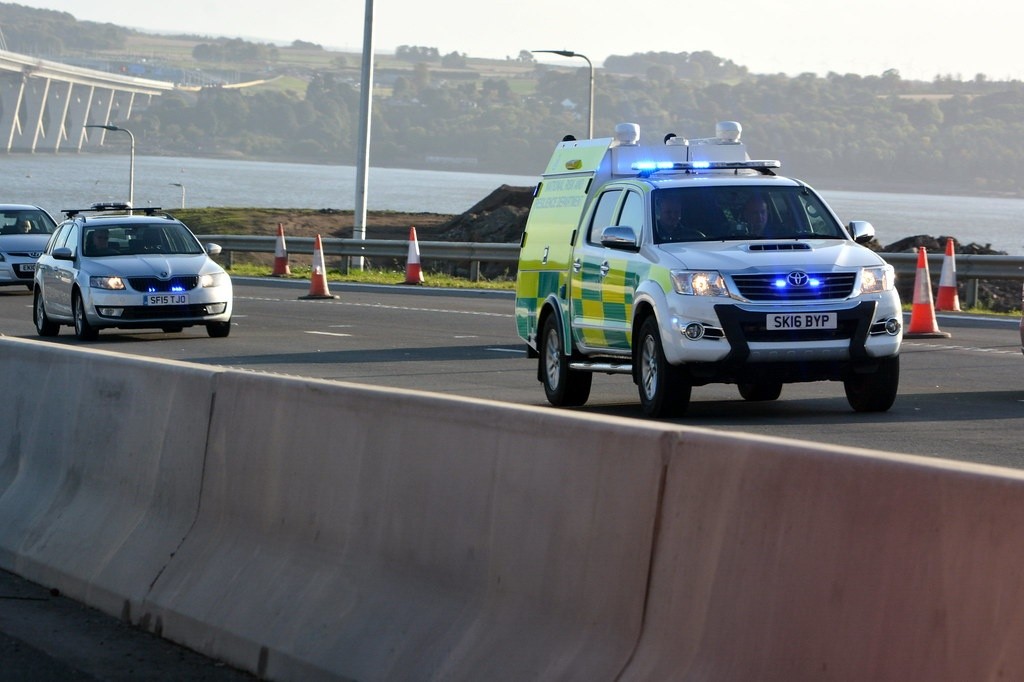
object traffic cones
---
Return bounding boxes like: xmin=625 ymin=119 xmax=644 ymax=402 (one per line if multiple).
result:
xmin=298 ymin=233 xmax=340 ymax=300
xmin=395 ymin=225 xmax=426 ymax=286
xmin=904 ymin=246 xmax=954 ymax=340
xmin=267 ymin=223 xmax=294 ymax=278
xmin=932 ymin=236 xmax=962 ymax=312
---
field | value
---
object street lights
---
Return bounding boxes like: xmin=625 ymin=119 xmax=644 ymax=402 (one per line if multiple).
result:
xmin=531 ymin=48 xmax=595 ymax=141
xmin=84 ymin=124 xmax=135 ymax=216
xmin=168 ymin=182 xmax=185 ymax=208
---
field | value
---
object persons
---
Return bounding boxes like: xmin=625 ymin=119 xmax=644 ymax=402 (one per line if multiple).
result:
xmin=92 ymin=229 xmax=112 ymax=249
xmin=653 ymin=194 xmax=706 ymax=241
xmin=17 ymin=218 xmax=33 ymax=233
xmin=736 ymin=195 xmax=772 ymax=238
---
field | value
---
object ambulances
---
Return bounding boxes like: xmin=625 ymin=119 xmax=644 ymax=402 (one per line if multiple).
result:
xmin=516 ymin=122 xmax=903 ymax=420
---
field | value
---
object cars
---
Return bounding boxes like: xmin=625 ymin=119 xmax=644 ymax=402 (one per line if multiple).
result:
xmin=32 ymin=201 xmax=234 ymax=342
xmin=0 ymin=203 xmax=60 ymax=293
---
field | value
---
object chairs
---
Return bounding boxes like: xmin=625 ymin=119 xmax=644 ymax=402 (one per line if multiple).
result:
xmin=86 ymin=230 xmax=115 ymax=257
xmin=128 ymin=237 xmax=158 ymax=253
